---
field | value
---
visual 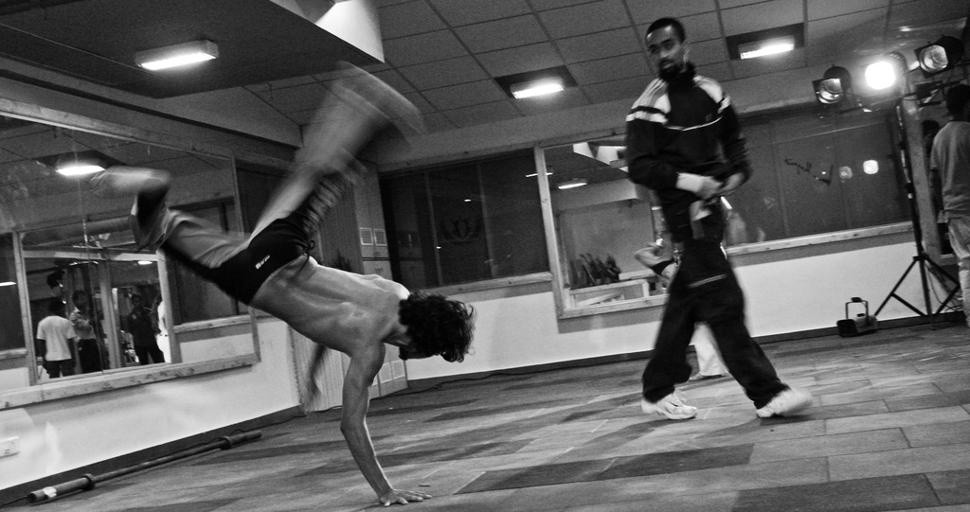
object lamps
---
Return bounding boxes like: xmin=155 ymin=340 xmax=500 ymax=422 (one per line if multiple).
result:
xmin=55 ymin=158 xmax=107 ymax=177
xmin=135 ymin=40 xmax=219 ymax=72
xmin=812 ymin=35 xmax=963 ymax=331
xmin=737 ymin=35 xmax=794 ymax=60
xmin=557 ymin=177 xmax=588 ymax=190
xmin=510 ymin=76 xmax=564 ymax=100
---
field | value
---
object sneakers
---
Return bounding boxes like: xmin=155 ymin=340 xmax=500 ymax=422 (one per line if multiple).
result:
xmin=755 ymin=388 xmax=814 ymax=418
xmin=687 ymin=371 xmax=723 ymax=381
xmin=640 ymin=389 xmax=698 ymax=420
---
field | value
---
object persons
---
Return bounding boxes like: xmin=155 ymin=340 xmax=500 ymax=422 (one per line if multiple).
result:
xmin=150 ymin=294 xmax=171 ymax=365
xmin=88 ymin=61 xmax=475 ymax=509
xmin=34 ymin=299 xmax=78 ymax=379
xmin=927 ymin=83 xmax=970 ymax=329
xmin=920 ymin=117 xmax=949 ymax=253
xmin=126 ymin=293 xmax=165 ymax=365
xmin=625 ymin=15 xmax=815 ymax=422
xmin=633 ymin=240 xmax=678 ymax=280
xmin=71 ymin=289 xmax=104 ymax=372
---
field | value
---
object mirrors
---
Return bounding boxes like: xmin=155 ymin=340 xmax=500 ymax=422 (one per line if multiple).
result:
xmin=0 ymin=97 xmax=261 ymax=411
xmin=532 ymin=125 xmax=671 ymax=320
